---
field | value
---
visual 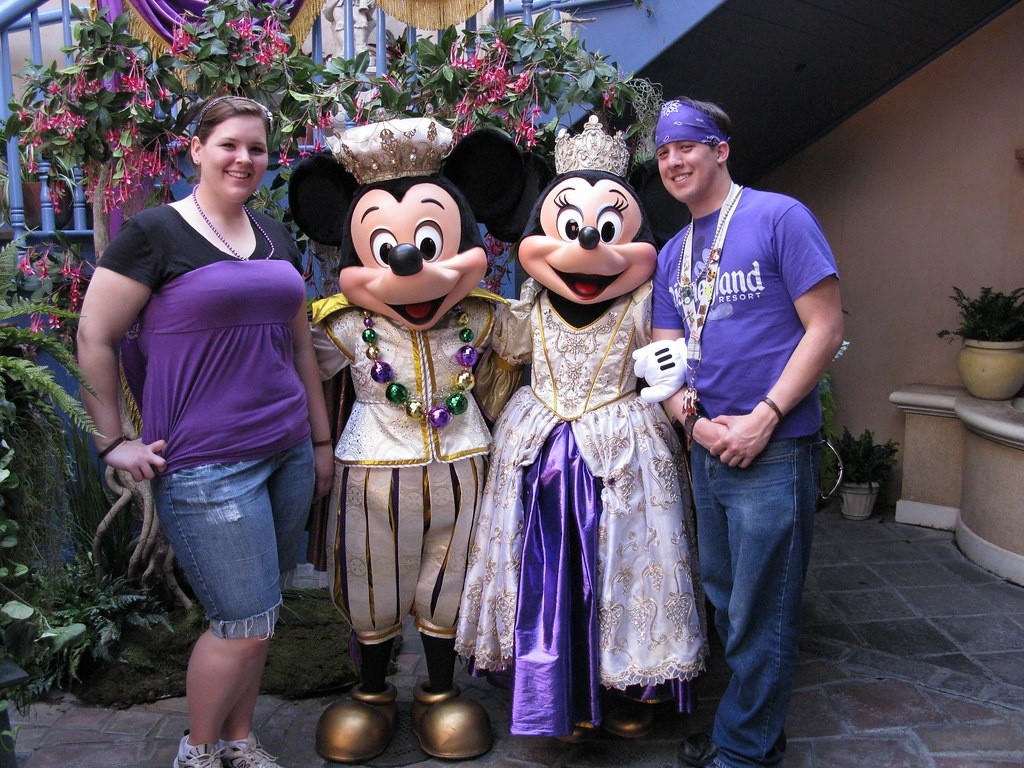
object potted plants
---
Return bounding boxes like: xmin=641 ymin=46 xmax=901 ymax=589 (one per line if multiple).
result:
xmin=936 ymin=286 xmax=1024 ymax=401
xmin=825 ymin=422 xmax=901 ymax=521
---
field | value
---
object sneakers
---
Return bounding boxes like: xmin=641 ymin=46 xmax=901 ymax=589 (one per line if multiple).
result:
xmin=675 ymin=724 xmax=787 ymax=767
xmin=173 ymin=729 xmax=281 ymax=768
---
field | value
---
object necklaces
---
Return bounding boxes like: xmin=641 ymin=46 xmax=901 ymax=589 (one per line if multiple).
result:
xmin=361 ymin=301 xmax=478 ymax=428
xmin=192 ymin=183 xmax=275 ymax=261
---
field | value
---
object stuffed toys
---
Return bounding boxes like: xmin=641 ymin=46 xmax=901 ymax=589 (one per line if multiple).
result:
xmin=287 ymin=118 xmax=528 ymax=764
xmin=455 ymin=116 xmax=711 ymax=746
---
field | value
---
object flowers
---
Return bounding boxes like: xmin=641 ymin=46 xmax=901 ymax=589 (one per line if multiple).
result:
xmin=0 ymin=0 xmax=667 ymax=365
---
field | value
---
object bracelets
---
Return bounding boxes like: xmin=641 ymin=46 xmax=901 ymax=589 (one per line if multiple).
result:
xmin=98 ymin=436 xmax=131 ymax=459
xmin=312 ymin=438 xmax=333 ymax=448
xmin=684 ymin=411 xmax=706 ymax=441
xmin=761 ymin=396 xmax=785 ymax=423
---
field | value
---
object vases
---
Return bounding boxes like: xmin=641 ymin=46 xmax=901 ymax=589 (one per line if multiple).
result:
xmin=20 ymin=181 xmax=74 ymax=231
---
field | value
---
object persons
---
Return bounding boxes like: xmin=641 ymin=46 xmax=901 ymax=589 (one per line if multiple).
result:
xmin=77 ymin=96 xmax=334 ymax=768
xmin=652 ymin=95 xmax=845 ymax=768
